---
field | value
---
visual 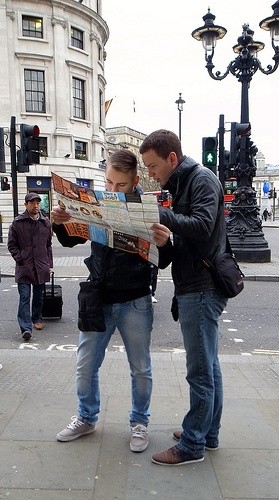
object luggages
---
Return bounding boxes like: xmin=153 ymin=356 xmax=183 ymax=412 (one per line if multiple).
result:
xmin=41 ymin=271 xmax=63 ymax=319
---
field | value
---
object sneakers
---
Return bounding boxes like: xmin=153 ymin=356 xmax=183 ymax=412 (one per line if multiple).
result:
xmin=130 ymin=422 xmax=149 ymax=452
xmin=56 ymin=415 xmax=96 ymax=442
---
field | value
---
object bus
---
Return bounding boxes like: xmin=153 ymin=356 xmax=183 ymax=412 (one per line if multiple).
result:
xmin=142 ymin=190 xmax=173 ymax=209
xmin=223 ymin=177 xmax=238 ymax=217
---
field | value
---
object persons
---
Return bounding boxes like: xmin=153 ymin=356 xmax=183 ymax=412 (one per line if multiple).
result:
xmin=50 ymin=149 xmax=175 ymax=451
xmin=264 ymin=208 xmax=269 ymax=221
xmin=8 ymin=192 xmax=53 ymax=338
xmin=139 ymin=129 xmax=226 ymax=466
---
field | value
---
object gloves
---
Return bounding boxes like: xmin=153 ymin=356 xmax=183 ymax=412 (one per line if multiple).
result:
xmin=170 ymin=296 xmax=179 ymax=322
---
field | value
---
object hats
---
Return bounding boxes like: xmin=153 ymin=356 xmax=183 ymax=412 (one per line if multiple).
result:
xmin=25 ymin=193 xmax=41 ymax=202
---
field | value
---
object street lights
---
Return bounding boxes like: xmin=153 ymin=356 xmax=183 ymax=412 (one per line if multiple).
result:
xmin=190 ymin=0 xmax=279 ymax=263
xmin=172 ymin=93 xmax=186 ymax=142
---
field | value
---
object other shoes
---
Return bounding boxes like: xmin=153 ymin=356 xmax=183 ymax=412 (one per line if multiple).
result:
xmin=22 ymin=331 xmax=32 ymax=341
xmin=173 ymin=431 xmax=219 ymax=451
xmin=151 ymin=445 xmax=204 ymax=466
xmin=34 ymin=322 xmax=44 ymax=330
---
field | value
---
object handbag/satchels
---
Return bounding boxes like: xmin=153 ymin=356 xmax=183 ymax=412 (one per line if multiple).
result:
xmin=75 ymin=281 xmax=107 ymax=333
xmin=209 ymin=254 xmax=244 ymax=298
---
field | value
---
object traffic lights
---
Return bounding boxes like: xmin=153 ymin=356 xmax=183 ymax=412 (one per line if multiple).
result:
xmin=201 ymin=136 xmax=218 ymax=175
xmin=228 ymin=122 xmax=251 ymax=166
xmin=268 ymin=189 xmax=273 ymax=199
xmin=1 ymin=176 xmax=11 ymax=192
xmin=19 ymin=123 xmax=41 ymax=166
